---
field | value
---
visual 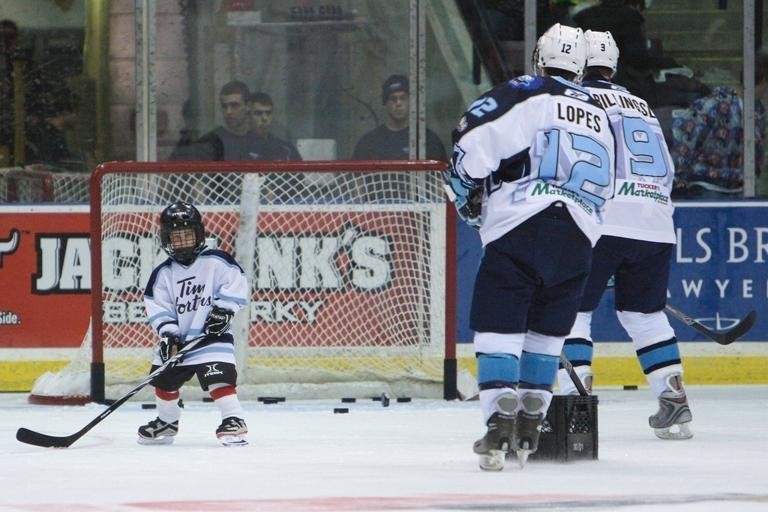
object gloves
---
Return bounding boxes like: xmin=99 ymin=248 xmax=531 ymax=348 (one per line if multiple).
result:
xmin=452 ymin=186 xmax=485 ymax=226
xmin=201 ymin=305 xmax=236 ymax=338
xmin=158 ymin=334 xmax=185 ymax=370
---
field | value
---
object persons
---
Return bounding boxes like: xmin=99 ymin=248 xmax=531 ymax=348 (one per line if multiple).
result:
xmin=191 ymin=83 xmax=276 ymax=206
xmin=442 ymin=22 xmax=615 ymax=479
xmin=352 ymin=73 xmax=449 ymax=205
xmin=132 ymin=200 xmax=253 ymax=447
xmin=481 ymin=0 xmax=768 ymax=204
xmin=0 ymin=19 xmax=88 ymax=173
xmin=248 ymin=96 xmax=307 ymax=204
xmin=556 ymin=22 xmax=697 ymax=444
xmin=166 ymin=100 xmax=224 ymax=207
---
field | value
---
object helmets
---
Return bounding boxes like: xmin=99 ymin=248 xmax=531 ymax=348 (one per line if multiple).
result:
xmin=381 ymin=73 xmax=410 ymax=106
xmin=583 ymin=28 xmax=622 ymax=77
xmin=156 ymin=202 xmax=205 ymax=264
xmin=530 ymin=21 xmax=583 ymax=78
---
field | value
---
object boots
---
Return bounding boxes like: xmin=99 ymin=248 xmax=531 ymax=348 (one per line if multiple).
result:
xmin=216 ymin=416 xmax=249 ymax=439
xmin=472 ymin=390 xmax=519 ymax=455
xmin=510 ymin=391 xmax=550 ymax=453
xmin=650 ymin=372 xmax=692 ymax=430
xmin=138 ymin=416 xmax=179 ymax=441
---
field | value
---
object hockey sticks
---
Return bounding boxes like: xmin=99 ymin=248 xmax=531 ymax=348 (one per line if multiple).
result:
xmin=666 ymin=307 xmax=757 ymax=344
xmin=16 ymin=329 xmax=212 ymax=447
